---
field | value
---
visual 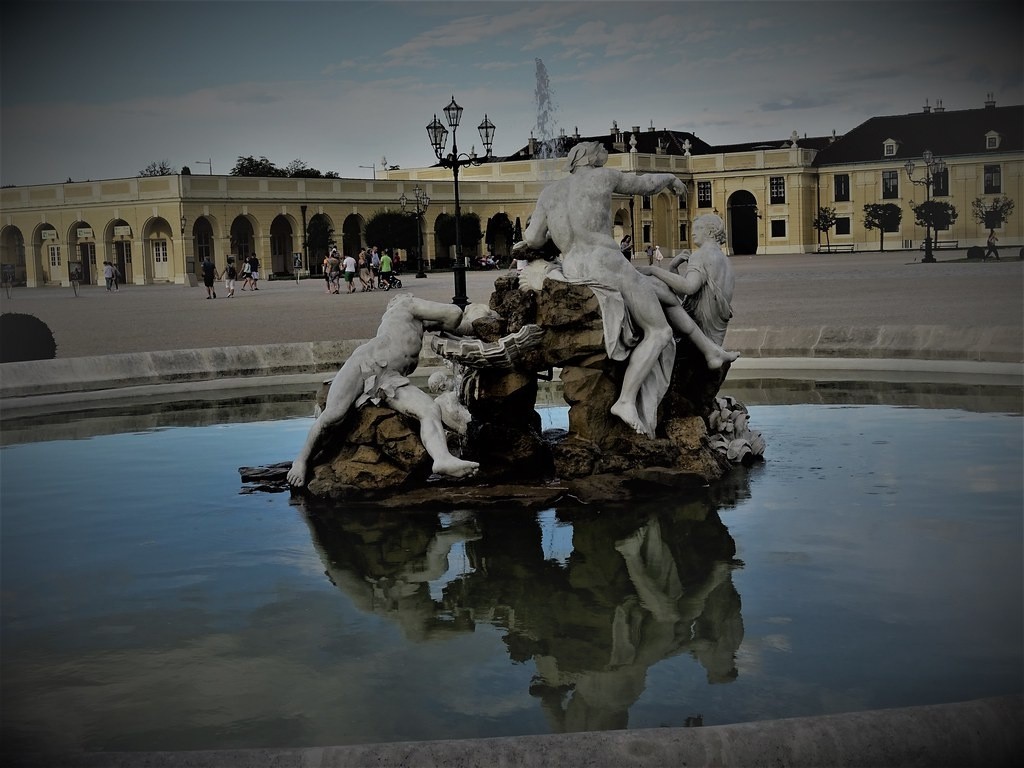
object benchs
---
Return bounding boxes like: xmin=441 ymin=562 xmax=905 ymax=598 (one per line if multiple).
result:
xmin=918 ymin=239 xmax=959 ymax=250
xmin=818 ymin=244 xmax=855 ymax=254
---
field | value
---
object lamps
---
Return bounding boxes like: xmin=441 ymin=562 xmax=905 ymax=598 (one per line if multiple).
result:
xmin=181 ymin=215 xmax=186 ymax=234
xmin=754 ymin=207 xmax=762 ymax=220
xmin=713 ymin=207 xmax=722 ymax=219
xmin=976 ymin=197 xmax=982 ymax=208
xmin=909 ymin=200 xmax=914 ymax=210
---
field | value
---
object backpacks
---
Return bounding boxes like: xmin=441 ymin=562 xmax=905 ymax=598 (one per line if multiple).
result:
xmin=226 ymin=264 xmax=235 ymax=278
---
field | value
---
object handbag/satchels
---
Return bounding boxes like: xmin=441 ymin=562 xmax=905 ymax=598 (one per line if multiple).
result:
xmin=115 ymin=269 xmax=120 ymax=277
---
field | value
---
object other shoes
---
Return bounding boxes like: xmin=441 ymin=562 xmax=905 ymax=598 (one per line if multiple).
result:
xmin=332 ymin=289 xmax=338 ymax=294
xmin=255 ymin=288 xmax=259 ymax=290
xmin=325 ymin=290 xmax=331 ymax=294
xmin=366 ymin=285 xmax=368 ymax=292
xmin=385 ymin=284 xmax=390 ymax=290
xmin=352 ymin=287 xmax=356 ymax=293
xmin=206 ymin=296 xmax=211 ymax=299
xmin=241 ymin=288 xmax=245 ymax=291
xmin=250 ymin=288 xmax=254 ymax=291
xmin=336 ymin=291 xmax=339 ymax=294
xmin=227 ymin=293 xmax=231 ymax=297
xmin=231 ymin=289 xmax=234 ymax=295
xmin=106 ymin=289 xmax=110 ymax=291
xmin=213 ymin=292 xmax=216 ymax=298
xmin=114 ymin=289 xmax=119 ymax=291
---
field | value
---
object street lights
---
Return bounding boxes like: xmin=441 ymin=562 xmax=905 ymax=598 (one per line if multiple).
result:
xmin=904 ymin=147 xmax=946 ymax=264
xmin=195 ymin=158 xmax=212 ymax=175
xmin=427 ymin=95 xmax=498 ymax=310
xmin=398 ymin=184 xmax=430 ymax=279
xmin=359 ymin=163 xmax=376 ymax=180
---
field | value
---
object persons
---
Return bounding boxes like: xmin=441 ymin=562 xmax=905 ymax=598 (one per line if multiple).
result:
xmin=481 ymin=255 xmax=501 ymax=270
xmin=238 ymin=252 xmax=260 ymax=291
xmin=981 ymin=231 xmax=1001 ymax=262
xmin=219 ymin=258 xmax=238 ymax=298
xmin=635 ymin=213 xmax=736 ymax=346
xmin=286 ymin=292 xmax=506 ymax=486
xmin=103 ymin=260 xmax=121 ymax=292
xmin=645 ymin=245 xmax=664 ymax=267
xmin=201 ymin=257 xmax=220 ymax=299
xmin=620 ymin=234 xmax=634 ymax=262
xmin=322 ymin=245 xmax=402 ymax=294
xmin=524 ymin=140 xmax=741 ymax=436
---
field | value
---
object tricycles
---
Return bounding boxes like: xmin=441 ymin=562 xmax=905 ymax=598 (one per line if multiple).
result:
xmin=377 ymin=268 xmax=402 ymax=289
